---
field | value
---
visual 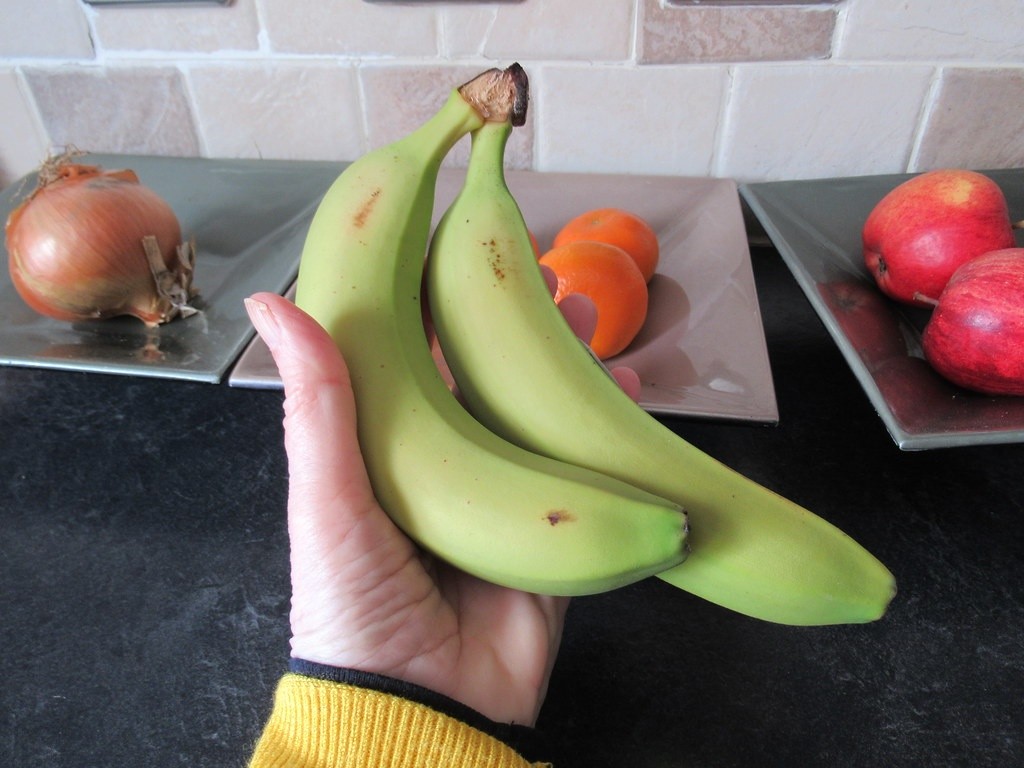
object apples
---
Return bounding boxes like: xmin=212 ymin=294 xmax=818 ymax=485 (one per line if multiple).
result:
xmin=818 ymin=278 xmax=944 ymax=432
xmin=864 ymin=169 xmax=1024 ymax=399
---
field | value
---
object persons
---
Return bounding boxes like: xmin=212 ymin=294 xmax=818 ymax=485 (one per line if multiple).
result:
xmin=240 ymin=253 xmax=641 ymax=768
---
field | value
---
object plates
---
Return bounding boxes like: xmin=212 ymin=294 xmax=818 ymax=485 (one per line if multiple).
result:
xmin=0 ymin=153 xmax=354 ymax=384
xmin=227 ymin=167 xmax=782 ymax=428
xmin=737 ymin=168 xmax=1023 ymax=451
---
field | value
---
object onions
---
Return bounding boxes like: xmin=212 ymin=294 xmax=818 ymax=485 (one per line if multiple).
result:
xmin=5 ymin=141 xmax=199 ymax=329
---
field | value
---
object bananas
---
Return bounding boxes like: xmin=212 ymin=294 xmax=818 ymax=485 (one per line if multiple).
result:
xmin=296 ymin=61 xmax=897 ymax=624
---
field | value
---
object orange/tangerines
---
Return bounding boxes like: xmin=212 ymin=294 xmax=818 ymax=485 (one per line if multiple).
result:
xmin=520 ymin=207 xmax=659 ymax=355
